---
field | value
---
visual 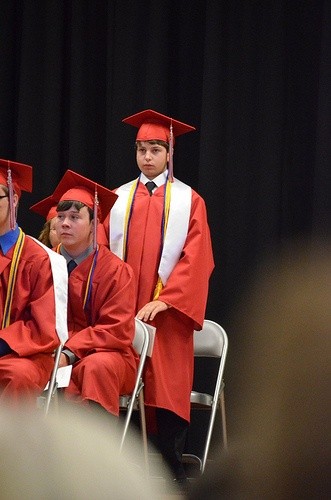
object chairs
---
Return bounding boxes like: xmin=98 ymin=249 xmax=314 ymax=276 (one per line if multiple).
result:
xmin=189 ymin=318 xmax=232 ymax=477
xmin=42 ymin=310 xmax=156 ymax=477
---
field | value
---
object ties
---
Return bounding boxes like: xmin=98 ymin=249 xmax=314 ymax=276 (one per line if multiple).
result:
xmin=68 ymin=259 xmax=77 ymax=279
xmin=145 ymin=181 xmax=156 ymax=197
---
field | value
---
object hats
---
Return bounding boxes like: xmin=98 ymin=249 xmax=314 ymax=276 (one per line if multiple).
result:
xmin=29 ymin=195 xmax=58 ymax=222
xmin=50 ymin=169 xmax=119 ymax=248
xmin=0 ymin=159 xmax=32 ymax=229
xmin=122 ymin=109 xmax=196 ymax=182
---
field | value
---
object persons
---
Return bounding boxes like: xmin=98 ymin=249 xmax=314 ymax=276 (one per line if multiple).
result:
xmin=49 ymin=168 xmax=137 ymax=428
xmin=100 ymin=109 xmax=215 ymax=496
xmin=29 ymin=193 xmax=60 ymax=249
xmin=0 ymin=158 xmax=59 ymax=434
